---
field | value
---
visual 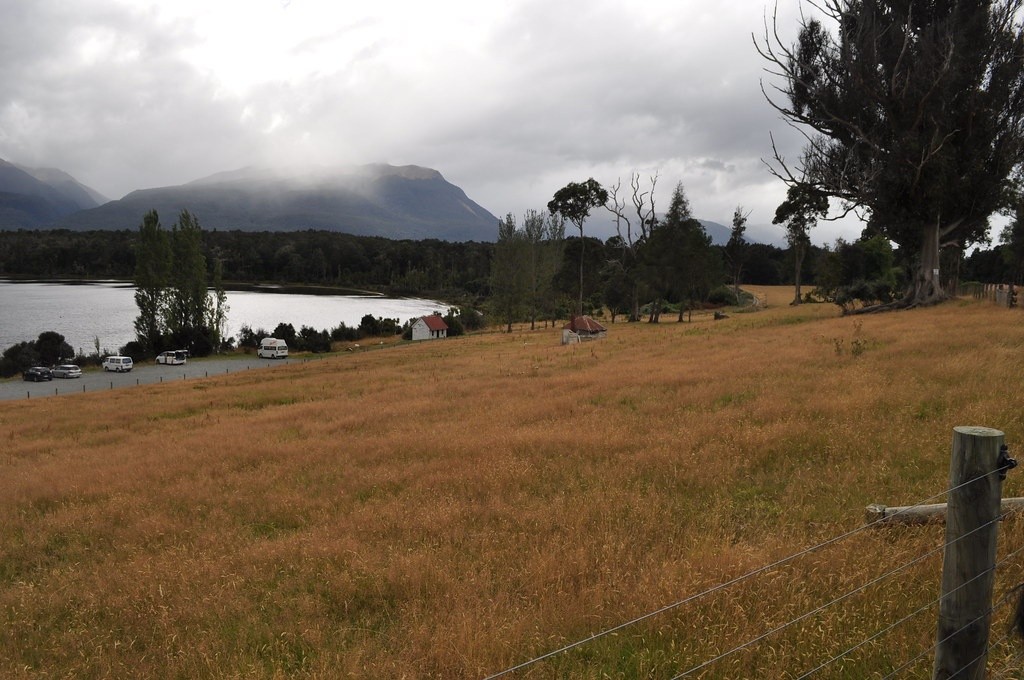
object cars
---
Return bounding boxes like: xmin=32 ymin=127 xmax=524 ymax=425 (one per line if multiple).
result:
xmin=49 ymin=364 xmax=82 ymax=379
xmin=22 ymin=367 xmax=52 ymax=382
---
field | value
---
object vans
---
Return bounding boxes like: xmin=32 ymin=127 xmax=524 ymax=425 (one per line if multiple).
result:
xmin=156 ymin=350 xmax=188 ymax=365
xmin=257 ymin=338 xmax=288 ymax=359
xmin=101 ymin=356 xmax=133 ymax=372
